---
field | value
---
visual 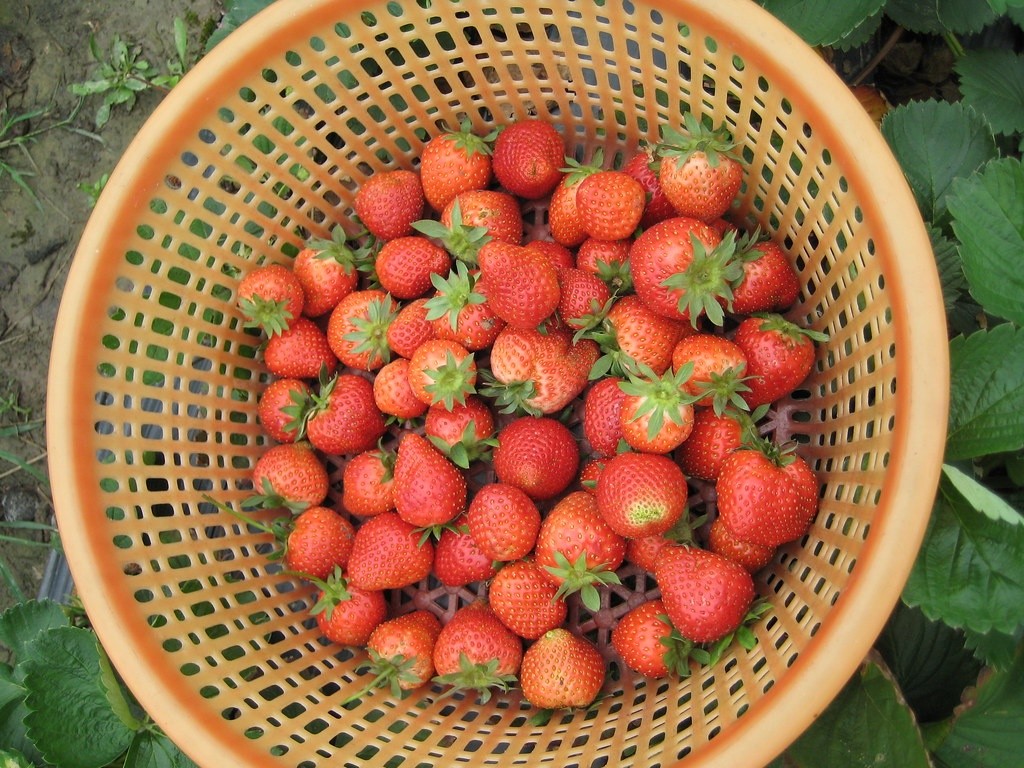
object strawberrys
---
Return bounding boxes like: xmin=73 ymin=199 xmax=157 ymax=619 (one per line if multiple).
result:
xmin=844 ymin=80 xmax=898 ymax=133
xmin=233 ymin=110 xmax=819 ymax=719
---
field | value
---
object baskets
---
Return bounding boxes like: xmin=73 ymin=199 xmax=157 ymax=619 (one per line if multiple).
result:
xmin=49 ymin=1 xmax=953 ymax=768
